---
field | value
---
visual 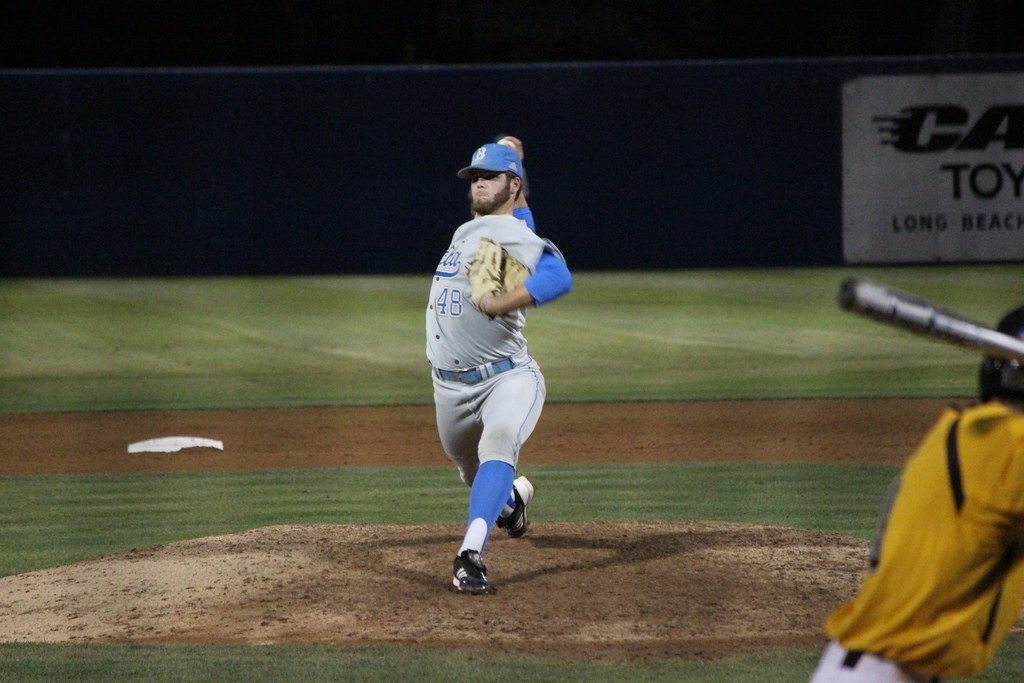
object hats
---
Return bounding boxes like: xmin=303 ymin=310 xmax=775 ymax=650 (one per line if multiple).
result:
xmin=458 ymin=143 xmax=523 ymax=180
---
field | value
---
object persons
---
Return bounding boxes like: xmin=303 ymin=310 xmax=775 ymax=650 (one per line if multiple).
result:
xmin=809 ymin=297 xmax=1024 ymax=683
xmin=426 ymin=135 xmax=574 ymax=594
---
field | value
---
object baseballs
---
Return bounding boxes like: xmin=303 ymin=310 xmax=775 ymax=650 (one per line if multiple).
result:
xmin=497 ymin=139 xmax=516 ymax=149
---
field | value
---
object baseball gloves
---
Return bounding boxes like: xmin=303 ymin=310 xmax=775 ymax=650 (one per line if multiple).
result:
xmin=463 ymin=237 xmax=533 ymax=321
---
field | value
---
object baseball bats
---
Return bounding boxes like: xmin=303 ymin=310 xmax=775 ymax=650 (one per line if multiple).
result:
xmin=834 ymin=278 xmax=1024 ymax=365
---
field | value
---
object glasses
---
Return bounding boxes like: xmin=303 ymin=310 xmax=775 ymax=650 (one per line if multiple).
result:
xmin=465 ymin=170 xmax=517 ymax=184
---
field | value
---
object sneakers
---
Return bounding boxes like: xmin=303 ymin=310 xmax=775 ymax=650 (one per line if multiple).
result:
xmin=452 ymin=548 xmax=493 ymax=594
xmin=496 ymin=476 xmax=534 ymax=539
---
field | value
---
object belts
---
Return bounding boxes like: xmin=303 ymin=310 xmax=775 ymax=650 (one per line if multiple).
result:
xmin=840 ymin=650 xmax=862 ymax=667
xmin=435 ymin=357 xmax=514 ymax=386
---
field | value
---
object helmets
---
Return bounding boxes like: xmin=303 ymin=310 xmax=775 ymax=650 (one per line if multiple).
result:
xmin=977 ymin=305 xmax=1024 ymax=406
